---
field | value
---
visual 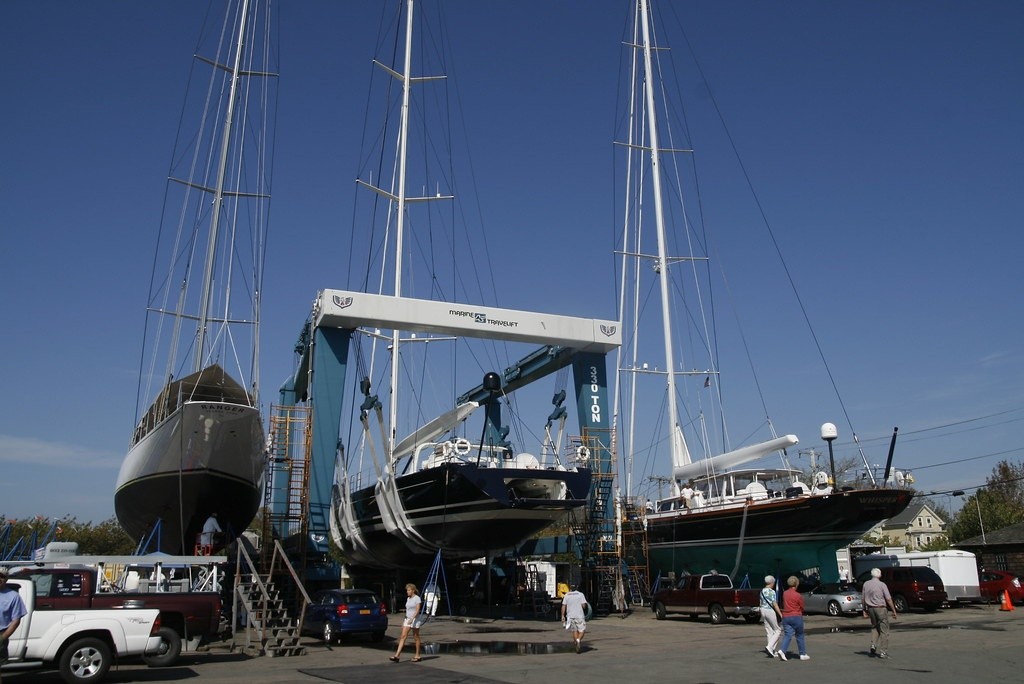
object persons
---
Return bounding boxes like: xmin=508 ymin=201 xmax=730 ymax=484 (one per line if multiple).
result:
xmin=390 ymin=583 xmax=424 ymax=663
xmin=778 ymin=575 xmax=811 ymax=660
xmin=839 ymin=565 xmax=848 ymax=582
xmin=681 ymin=482 xmax=695 ymax=500
xmin=861 ymin=567 xmax=898 ymax=659
xmin=759 ymin=574 xmax=783 ymax=658
xmin=645 ymin=499 xmax=654 ymax=514
xmin=560 ymin=581 xmax=587 ymax=655
xmin=197 ymin=512 xmax=222 ymax=556
xmin=423 ymin=578 xmax=442 ymax=616
xmin=0 ymin=566 xmax=27 ymax=665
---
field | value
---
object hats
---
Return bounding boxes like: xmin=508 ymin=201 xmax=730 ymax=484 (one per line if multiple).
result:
xmin=0 ymin=566 xmax=9 ymax=578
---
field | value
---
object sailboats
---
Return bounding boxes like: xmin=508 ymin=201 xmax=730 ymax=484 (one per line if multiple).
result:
xmin=112 ymin=0 xmax=914 ymax=584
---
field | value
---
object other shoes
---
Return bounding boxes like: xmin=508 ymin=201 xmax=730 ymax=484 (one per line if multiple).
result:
xmin=390 ymin=656 xmax=400 ymax=662
xmin=879 ymin=651 xmax=888 ymax=658
xmin=765 ymin=646 xmax=779 ymax=658
xmin=870 ymin=645 xmax=876 ymax=653
xmin=576 ymin=639 xmax=581 ymax=652
xmin=410 ymin=657 xmax=422 ymax=662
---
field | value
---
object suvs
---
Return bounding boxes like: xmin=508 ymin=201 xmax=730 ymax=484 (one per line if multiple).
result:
xmin=850 ymin=567 xmax=947 ymax=613
xmin=296 ymin=589 xmax=388 ymax=645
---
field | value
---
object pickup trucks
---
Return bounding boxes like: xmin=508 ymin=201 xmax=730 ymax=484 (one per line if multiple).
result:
xmin=651 ymin=570 xmax=764 ymax=623
xmin=0 ymin=568 xmax=223 ymax=684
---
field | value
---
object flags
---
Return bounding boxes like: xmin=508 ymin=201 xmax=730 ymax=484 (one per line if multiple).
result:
xmin=704 ymin=376 xmax=710 ymax=387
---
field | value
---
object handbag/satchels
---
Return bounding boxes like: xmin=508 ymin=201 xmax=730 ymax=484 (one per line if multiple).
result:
xmin=775 ymin=608 xmax=782 ymax=623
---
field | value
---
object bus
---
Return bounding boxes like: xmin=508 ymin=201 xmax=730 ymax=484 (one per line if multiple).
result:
xmin=850 ymin=549 xmax=980 ymax=608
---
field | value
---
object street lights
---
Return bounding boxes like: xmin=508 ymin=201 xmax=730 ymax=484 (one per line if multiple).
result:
xmin=953 ymin=489 xmax=985 ymax=543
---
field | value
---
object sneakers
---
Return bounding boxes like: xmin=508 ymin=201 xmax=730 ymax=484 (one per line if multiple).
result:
xmin=777 ymin=649 xmax=787 ymax=661
xmin=799 ymin=654 xmax=811 ymax=661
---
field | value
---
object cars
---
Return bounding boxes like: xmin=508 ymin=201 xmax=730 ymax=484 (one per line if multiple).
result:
xmin=799 ymin=584 xmax=868 ymax=617
xmin=979 ymin=570 xmax=1024 ymax=604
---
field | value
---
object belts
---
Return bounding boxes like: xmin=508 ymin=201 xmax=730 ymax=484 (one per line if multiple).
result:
xmin=867 ymin=605 xmax=886 ymax=608
